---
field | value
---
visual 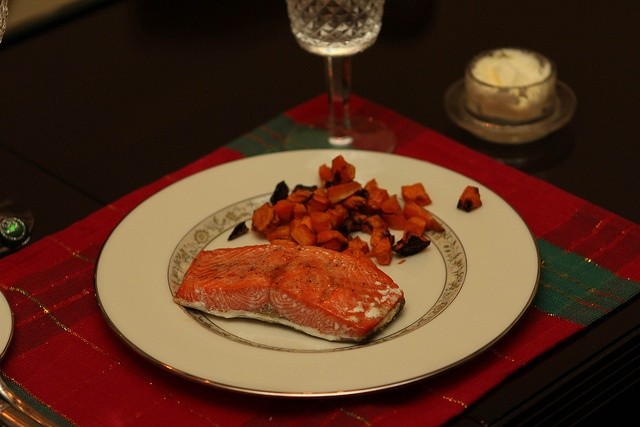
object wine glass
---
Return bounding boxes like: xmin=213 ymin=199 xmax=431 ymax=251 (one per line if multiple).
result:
xmin=280 ymin=0 xmax=398 ymax=153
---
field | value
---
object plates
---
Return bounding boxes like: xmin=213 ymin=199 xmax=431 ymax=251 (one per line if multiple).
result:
xmin=0 ymin=291 xmax=15 ymax=360
xmin=91 ymin=147 xmax=542 ymax=398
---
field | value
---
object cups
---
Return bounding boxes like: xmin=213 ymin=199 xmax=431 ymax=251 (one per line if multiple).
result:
xmin=462 ymin=46 xmax=557 ymax=125
